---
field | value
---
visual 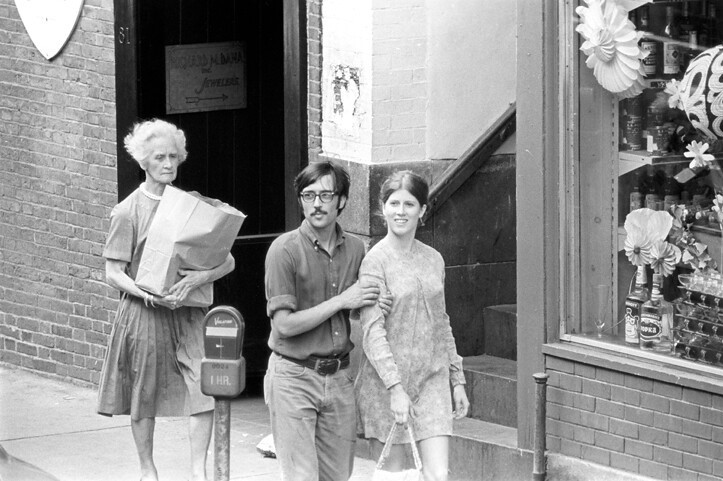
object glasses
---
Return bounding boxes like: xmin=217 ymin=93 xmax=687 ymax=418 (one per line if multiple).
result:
xmin=298 ymin=190 xmax=339 ymax=203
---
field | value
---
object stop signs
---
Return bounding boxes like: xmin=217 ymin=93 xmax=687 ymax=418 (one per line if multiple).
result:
xmin=202 ymin=305 xmax=244 ymax=360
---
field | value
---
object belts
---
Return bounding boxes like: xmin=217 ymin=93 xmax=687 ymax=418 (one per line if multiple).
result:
xmin=282 ymin=352 xmax=350 ymax=377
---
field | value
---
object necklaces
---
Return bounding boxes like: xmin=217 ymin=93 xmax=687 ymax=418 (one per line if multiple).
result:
xmin=139 ymin=180 xmax=173 ymax=201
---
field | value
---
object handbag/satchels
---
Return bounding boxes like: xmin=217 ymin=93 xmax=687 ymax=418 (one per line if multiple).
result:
xmin=348 ymin=419 xmax=424 ymax=481
xmin=135 ymin=185 xmax=248 ymax=308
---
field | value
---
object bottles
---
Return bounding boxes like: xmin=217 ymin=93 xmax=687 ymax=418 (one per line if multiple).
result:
xmin=639 ymin=274 xmax=672 ymax=351
xmin=618 ymin=0 xmax=723 ymax=152
xmin=627 ymin=165 xmax=718 ymax=228
xmin=625 ymin=263 xmax=651 ymax=345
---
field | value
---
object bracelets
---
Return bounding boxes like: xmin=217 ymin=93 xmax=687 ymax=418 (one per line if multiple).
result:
xmin=143 ymin=292 xmax=159 ymax=308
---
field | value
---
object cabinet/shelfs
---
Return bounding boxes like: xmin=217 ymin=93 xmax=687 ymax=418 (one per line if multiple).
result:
xmin=581 ymin=0 xmax=723 ymax=337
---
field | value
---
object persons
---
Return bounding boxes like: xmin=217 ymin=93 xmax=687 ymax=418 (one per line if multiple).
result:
xmin=102 ymin=117 xmax=237 ymax=481
xmin=359 ymin=169 xmax=471 ymax=481
xmin=263 ymin=160 xmax=394 ymax=481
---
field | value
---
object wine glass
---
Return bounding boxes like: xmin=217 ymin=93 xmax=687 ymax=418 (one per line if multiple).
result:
xmin=673 ymin=273 xmax=723 ymax=366
xmin=590 ymin=284 xmax=609 ymax=342
